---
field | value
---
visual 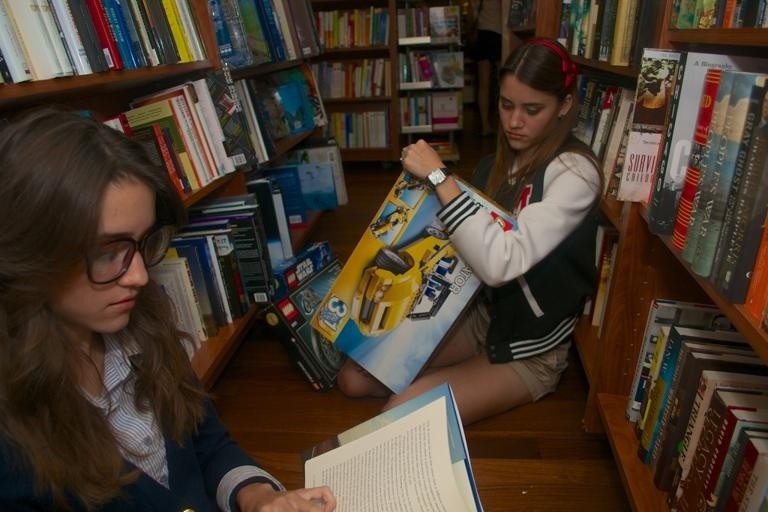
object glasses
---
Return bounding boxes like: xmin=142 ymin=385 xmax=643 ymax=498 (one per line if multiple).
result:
xmin=84 ymin=224 xmax=174 ymax=286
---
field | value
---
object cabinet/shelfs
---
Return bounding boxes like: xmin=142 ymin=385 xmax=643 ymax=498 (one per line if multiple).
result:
xmin=308 ymin=0 xmax=466 ymax=167
xmin=497 ymin=1 xmax=767 ymax=510
xmin=2 ymin=0 xmax=336 ymax=395
